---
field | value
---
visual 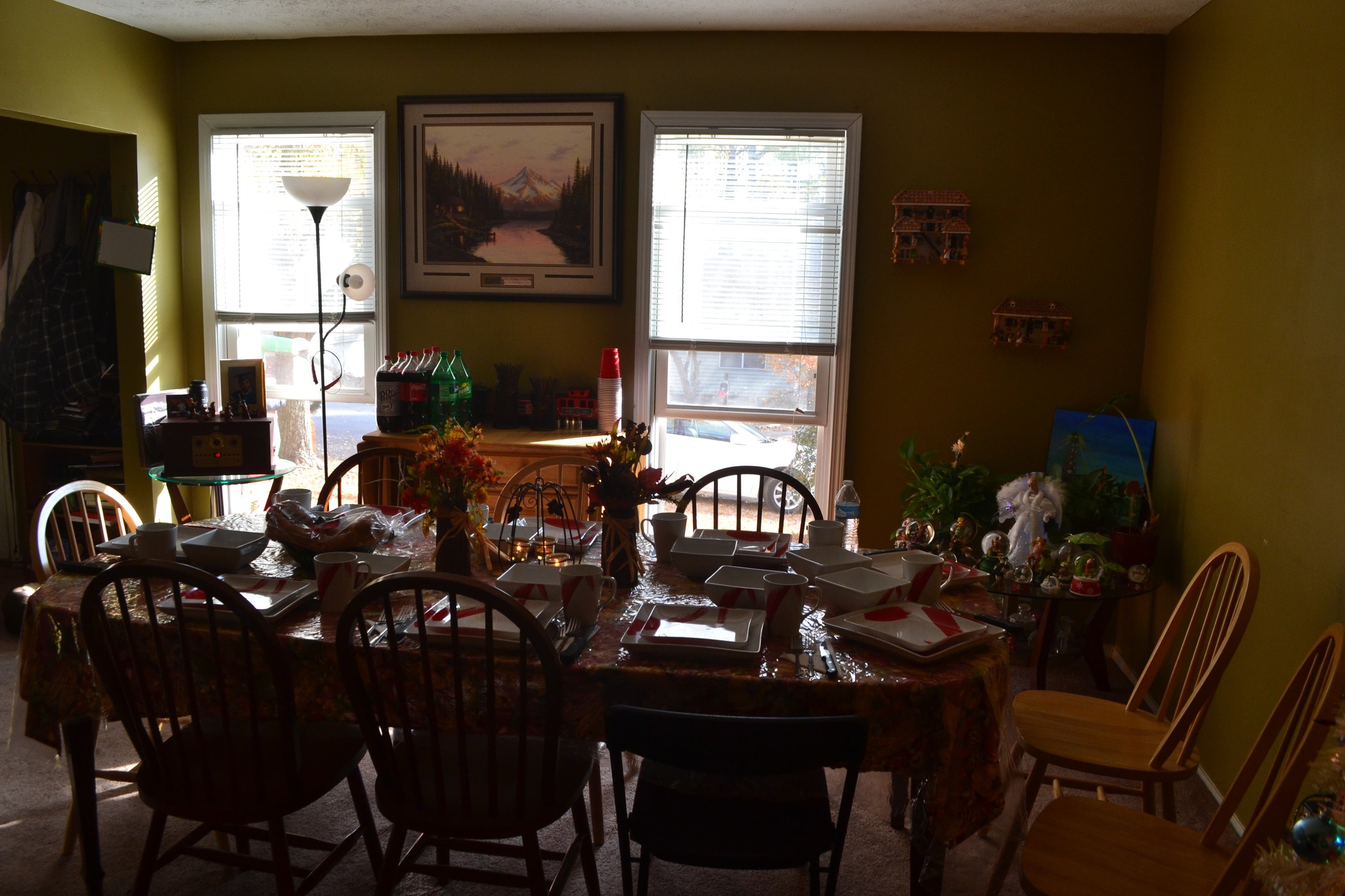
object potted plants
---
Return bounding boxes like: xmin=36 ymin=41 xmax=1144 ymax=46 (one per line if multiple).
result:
xmin=1078 ymin=391 xmax=1162 ymax=568
xmin=895 ymin=431 xmax=1019 ymax=547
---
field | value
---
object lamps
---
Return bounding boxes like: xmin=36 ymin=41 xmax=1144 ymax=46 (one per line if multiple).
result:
xmin=281 ymin=174 xmax=376 ymax=482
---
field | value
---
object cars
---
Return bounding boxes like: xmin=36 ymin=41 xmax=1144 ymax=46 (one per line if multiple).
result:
xmin=654 ymin=403 xmax=817 ymax=515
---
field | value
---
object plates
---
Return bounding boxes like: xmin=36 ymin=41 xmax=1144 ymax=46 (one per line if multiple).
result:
xmin=95 ymin=512 xmax=1008 ymax=665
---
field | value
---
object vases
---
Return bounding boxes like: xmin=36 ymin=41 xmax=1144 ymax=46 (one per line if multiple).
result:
xmin=433 ymin=498 xmax=476 ymax=578
xmin=602 ymin=499 xmax=646 ymax=592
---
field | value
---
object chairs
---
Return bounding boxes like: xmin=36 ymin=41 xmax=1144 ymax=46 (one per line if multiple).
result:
xmin=1015 ymin=622 xmax=1345 ymax=895
xmin=493 ymin=457 xmax=650 ymax=849
xmin=601 ymin=697 xmax=869 ymax=895
xmin=333 ymin=570 xmax=605 ymax=895
xmin=29 ymin=480 xmax=237 ymax=856
xmin=971 ymin=541 xmax=1262 ymax=895
xmin=314 ymin=442 xmax=476 ymax=527
xmin=78 ymin=554 xmax=387 ymax=896
xmin=673 ymin=464 xmax=826 ymax=542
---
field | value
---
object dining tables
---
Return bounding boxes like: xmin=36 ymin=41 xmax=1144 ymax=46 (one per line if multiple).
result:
xmin=10 ymin=522 xmax=1015 ymax=896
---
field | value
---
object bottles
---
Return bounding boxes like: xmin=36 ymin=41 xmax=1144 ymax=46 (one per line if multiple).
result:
xmin=376 ymin=347 xmax=473 ymax=432
xmin=835 ymin=480 xmax=859 ymax=553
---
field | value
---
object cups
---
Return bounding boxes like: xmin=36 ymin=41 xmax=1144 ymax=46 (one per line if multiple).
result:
xmin=270 ymin=489 xmax=312 ymax=512
xmin=597 ymin=347 xmax=623 ymax=434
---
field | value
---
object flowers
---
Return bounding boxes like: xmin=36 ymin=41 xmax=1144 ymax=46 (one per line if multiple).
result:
xmin=396 ymin=415 xmax=504 ymax=533
xmin=579 ymin=416 xmax=696 ymax=517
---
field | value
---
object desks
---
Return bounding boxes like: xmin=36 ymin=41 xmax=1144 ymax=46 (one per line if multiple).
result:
xmin=920 ymin=539 xmax=1166 ymax=693
xmin=150 ymin=456 xmax=299 ymax=524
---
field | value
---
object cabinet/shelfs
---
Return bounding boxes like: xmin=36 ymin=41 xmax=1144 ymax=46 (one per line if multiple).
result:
xmin=16 ymin=431 xmax=126 ymax=580
xmin=362 ymin=426 xmax=646 ymax=534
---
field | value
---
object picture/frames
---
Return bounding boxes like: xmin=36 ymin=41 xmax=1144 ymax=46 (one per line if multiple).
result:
xmin=220 ymin=358 xmax=268 ymax=418
xmin=396 ymin=92 xmax=625 ymax=306
